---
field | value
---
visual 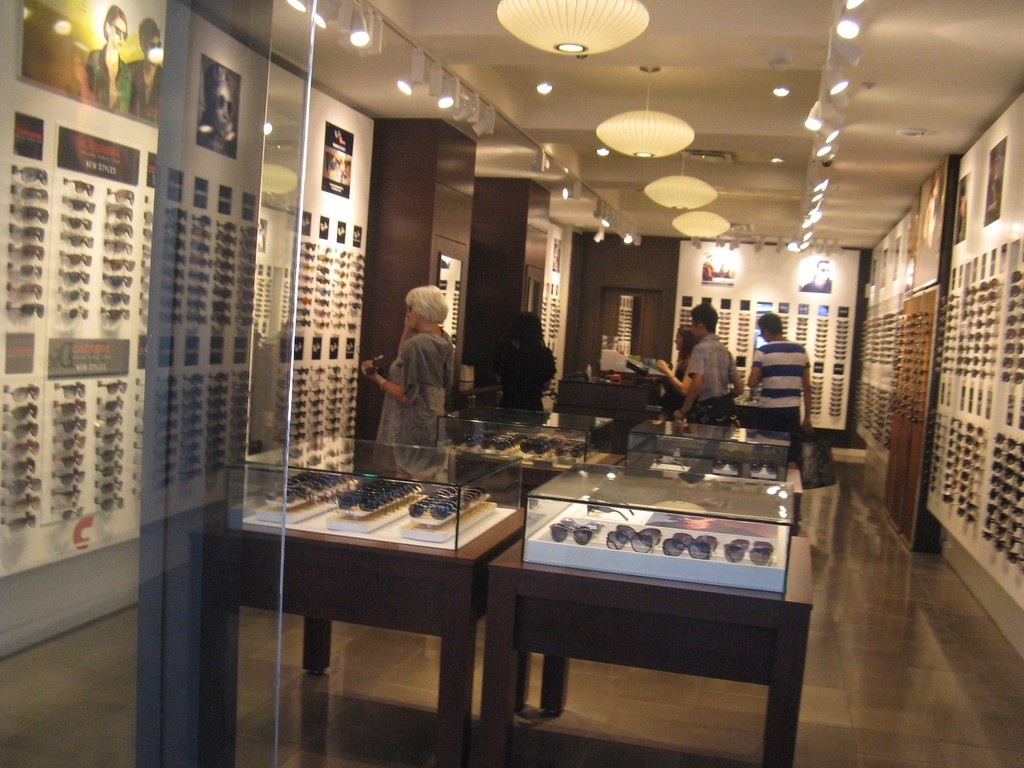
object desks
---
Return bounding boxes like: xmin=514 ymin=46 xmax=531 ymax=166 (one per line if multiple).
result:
xmin=203 ymin=370 xmax=812 ymax=765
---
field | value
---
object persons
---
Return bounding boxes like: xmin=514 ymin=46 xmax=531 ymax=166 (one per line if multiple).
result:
xmin=657 ymin=325 xmax=693 ymax=421
xmin=361 ymin=285 xmax=452 ymax=478
xmin=198 ymin=66 xmax=233 ymax=152
xmin=496 ymin=312 xmax=556 ymax=411
xmin=703 ymin=255 xmax=715 ymax=281
xmin=801 ymin=260 xmax=831 ymax=293
xmin=747 ymin=316 xmax=814 ymax=467
xmin=675 ymin=305 xmax=744 ymax=427
xmin=74 ymin=7 xmax=163 ymax=122
xmin=328 ymin=155 xmax=350 ymax=173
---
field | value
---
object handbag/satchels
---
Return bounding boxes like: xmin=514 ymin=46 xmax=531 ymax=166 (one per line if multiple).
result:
xmin=694 ymin=392 xmax=738 ymax=426
xmin=800 ymin=431 xmax=838 ymax=489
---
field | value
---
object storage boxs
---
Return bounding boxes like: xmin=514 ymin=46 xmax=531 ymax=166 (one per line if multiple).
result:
xmin=239 ymin=436 xmax=520 ymax=552
xmin=523 ymin=462 xmax=797 ymax=593
xmin=626 ymin=418 xmax=792 ymax=484
xmin=438 ymin=407 xmax=615 ymax=472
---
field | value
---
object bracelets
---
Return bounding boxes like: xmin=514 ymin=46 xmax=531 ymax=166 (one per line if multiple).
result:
xmin=379 ymin=379 xmax=386 ymax=391
xmin=679 ymin=409 xmax=686 ymax=415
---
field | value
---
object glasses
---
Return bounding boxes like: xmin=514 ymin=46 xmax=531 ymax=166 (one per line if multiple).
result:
xmin=1 ymin=165 xmax=256 ymax=532
xmin=454 ymin=421 xmax=592 ymax=459
xmin=656 ymin=440 xmax=787 ymax=478
xmin=539 ymin=295 xmax=563 ymax=340
xmin=265 ymin=466 xmax=489 ymax=520
xmin=850 ymin=271 xmax=1023 ymax=565
xmin=364 ymin=366 xmax=385 ymax=376
xmin=603 ymin=296 xmax=850 ymax=416
xmin=408 ymin=305 xmax=414 ymax=312
xmin=551 ymin=516 xmax=774 ymax=567
xmin=445 ymin=292 xmax=461 ymax=338
xmin=287 ymin=236 xmax=366 ymax=468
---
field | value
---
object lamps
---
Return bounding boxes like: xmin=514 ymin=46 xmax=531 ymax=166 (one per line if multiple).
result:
xmin=283 ymin=1 xmax=869 ymax=250
